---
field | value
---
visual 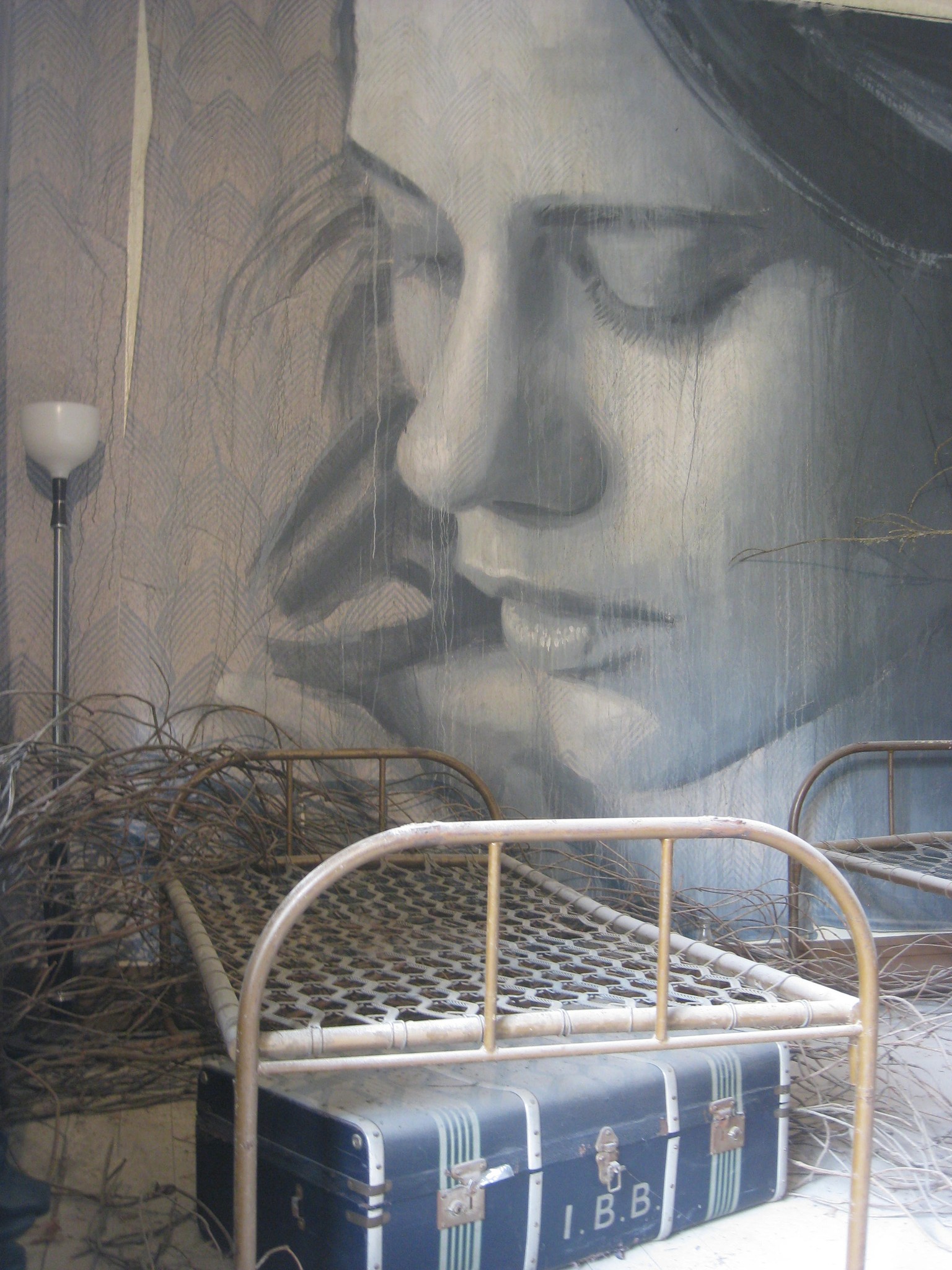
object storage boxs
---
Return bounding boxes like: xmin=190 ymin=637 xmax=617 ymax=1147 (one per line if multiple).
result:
xmin=193 ymin=1026 xmax=788 ymax=1270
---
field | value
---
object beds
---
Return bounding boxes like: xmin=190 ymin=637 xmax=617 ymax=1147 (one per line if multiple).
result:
xmin=785 ymin=740 xmax=952 ymax=981
xmin=157 ymin=746 xmax=883 ymax=1270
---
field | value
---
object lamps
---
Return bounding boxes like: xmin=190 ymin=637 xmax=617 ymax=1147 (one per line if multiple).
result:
xmin=21 ymin=396 xmax=104 ymax=983
